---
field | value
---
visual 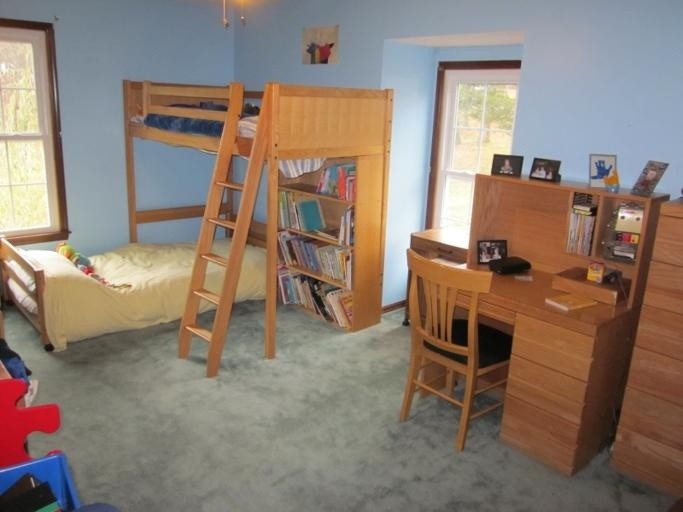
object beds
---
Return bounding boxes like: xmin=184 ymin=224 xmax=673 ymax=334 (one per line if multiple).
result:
xmin=0 ymin=234 xmax=268 ymax=353
xmin=122 ymin=79 xmax=394 ymax=161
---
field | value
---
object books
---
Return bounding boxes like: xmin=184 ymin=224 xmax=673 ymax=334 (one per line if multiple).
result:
xmin=543 ymin=293 xmax=598 ymax=313
xmin=276 ymin=156 xmax=357 ymax=334
xmin=565 ymin=201 xmax=597 ymax=258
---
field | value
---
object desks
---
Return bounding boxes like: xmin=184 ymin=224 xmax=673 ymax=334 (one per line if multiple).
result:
xmin=417 ymin=172 xmax=670 ymax=478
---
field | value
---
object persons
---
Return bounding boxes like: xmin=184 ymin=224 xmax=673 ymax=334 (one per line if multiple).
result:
xmin=479 ymin=243 xmax=502 ymax=262
xmin=499 ymin=158 xmax=513 ymax=173
xmin=531 ymin=164 xmax=552 ymax=181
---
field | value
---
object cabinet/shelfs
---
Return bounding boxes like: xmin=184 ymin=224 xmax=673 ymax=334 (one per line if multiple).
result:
xmin=401 ymin=222 xmax=469 ymax=325
xmin=607 ymin=196 xmax=682 ymax=499
xmin=276 ymin=155 xmax=381 ymax=332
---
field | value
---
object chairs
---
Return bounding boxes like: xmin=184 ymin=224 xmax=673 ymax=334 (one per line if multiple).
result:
xmin=399 ymin=248 xmax=510 ymax=452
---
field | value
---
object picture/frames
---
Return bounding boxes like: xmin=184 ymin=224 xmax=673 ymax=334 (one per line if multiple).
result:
xmin=490 ymin=153 xmax=523 ymax=177
xmin=588 ymin=154 xmax=617 ymax=190
xmin=528 ymin=157 xmax=562 ymax=183
xmin=476 ymin=240 xmax=509 ymax=265
xmin=630 ymin=160 xmax=669 ymax=198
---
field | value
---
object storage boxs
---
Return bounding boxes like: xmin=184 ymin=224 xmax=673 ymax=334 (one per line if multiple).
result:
xmin=1 ymin=450 xmax=81 ymax=512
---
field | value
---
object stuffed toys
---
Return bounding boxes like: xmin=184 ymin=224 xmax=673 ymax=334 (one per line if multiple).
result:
xmin=56 ymin=240 xmax=107 ymax=286
